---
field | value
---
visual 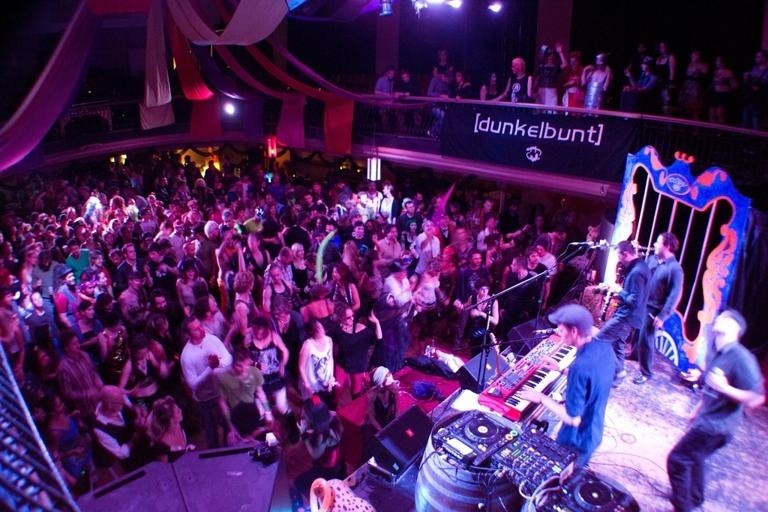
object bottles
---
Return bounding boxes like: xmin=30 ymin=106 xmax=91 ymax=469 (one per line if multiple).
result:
xmin=431 ymin=336 xmax=437 ymax=358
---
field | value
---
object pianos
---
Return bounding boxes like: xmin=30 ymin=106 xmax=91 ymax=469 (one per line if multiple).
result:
xmin=478 ymin=334 xmax=577 ymax=421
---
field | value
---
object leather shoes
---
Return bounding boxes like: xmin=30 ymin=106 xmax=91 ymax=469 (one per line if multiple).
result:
xmin=634 ymin=376 xmax=648 ymax=384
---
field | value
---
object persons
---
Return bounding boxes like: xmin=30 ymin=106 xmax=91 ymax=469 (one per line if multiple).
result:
xmin=2 ymin=156 xmax=623 ymax=461
xmin=597 ymin=242 xmax=652 ymax=379
xmin=376 ymin=41 xmax=768 ymax=137
xmin=624 ymin=233 xmax=685 ymax=384
xmin=666 ymin=308 xmax=767 ymax=512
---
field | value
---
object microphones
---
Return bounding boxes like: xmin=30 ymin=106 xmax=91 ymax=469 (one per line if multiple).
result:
xmin=570 ymin=239 xmax=609 ymax=250
xmin=648 ymin=313 xmax=665 ymax=331
xmin=532 ymin=328 xmax=558 ymax=335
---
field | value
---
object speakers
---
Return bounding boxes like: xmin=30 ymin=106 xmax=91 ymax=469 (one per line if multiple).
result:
xmin=507 ymin=318 xmax=553 ymax=351
xmin=374 ymin=404 xmax=437 ymax=476
xmin=456 ymin=347 xmax=511 ymax=393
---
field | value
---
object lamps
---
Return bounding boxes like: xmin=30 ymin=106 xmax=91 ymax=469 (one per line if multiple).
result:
xmin=366 ymin=157 xmax=382 ymax=182
xmin=378 ymin=0 xmax=429 ymax=19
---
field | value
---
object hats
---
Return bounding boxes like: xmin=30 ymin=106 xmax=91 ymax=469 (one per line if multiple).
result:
xmin=548 ymin=304 xmax=593 ymax=327
xmin=56 ymin=266 xmax=73 ymax=278
